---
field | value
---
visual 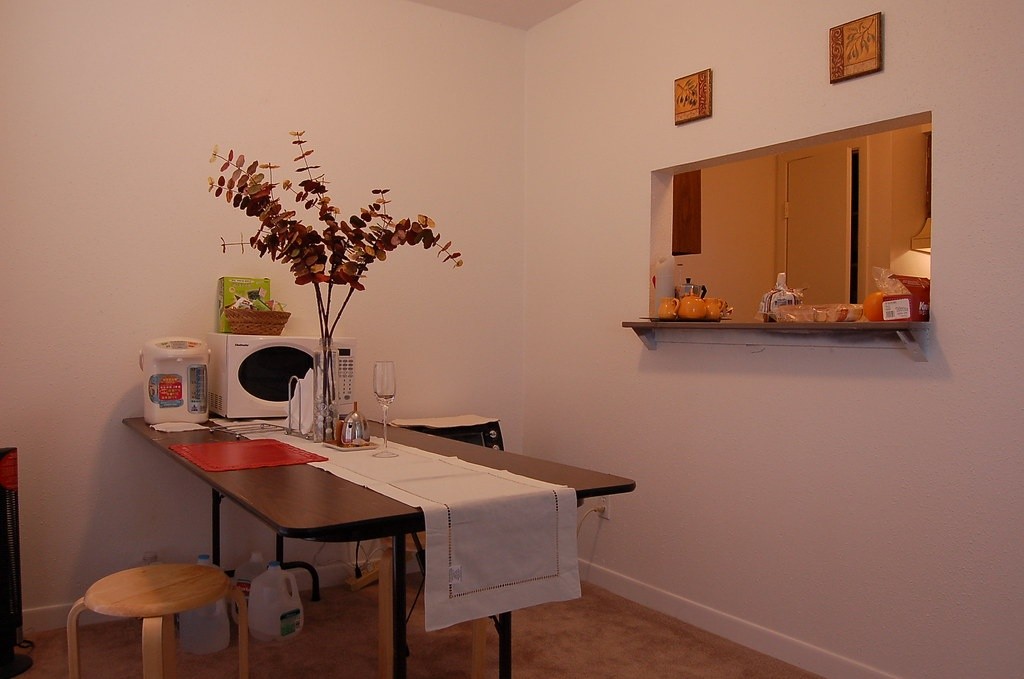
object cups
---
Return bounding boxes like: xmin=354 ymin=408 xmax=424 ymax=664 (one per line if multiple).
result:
xmin=658 ymin=292 xmax=724 ymax=321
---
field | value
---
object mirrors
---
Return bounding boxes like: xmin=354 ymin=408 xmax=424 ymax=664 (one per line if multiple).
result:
xmin=647 ymin=109 xmax=933 ymax=324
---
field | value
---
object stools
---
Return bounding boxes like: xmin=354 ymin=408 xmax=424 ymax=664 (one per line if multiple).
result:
xmin=65 ymin=563 xmax=249 ymax=679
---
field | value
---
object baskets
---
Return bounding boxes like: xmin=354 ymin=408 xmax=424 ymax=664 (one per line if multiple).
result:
xmin=224 ymin=302 xmax=291 ymax=335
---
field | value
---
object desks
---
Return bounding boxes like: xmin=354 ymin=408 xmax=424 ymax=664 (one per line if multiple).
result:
xmin=120 ymin=410 xmax=637 ymax=679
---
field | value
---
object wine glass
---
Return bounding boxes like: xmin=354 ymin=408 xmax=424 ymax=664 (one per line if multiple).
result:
xmin=372 ymin=361 xmax=399 ymax=458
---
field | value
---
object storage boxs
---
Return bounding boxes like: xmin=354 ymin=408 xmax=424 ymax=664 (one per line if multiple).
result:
xmin=217 ymin=276 xmax=271 ymax=333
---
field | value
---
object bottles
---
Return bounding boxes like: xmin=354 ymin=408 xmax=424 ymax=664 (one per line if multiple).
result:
xmin=180 ymin=554 xmax=230 ymax=653
xmin=232 ymin=553 xmax=268 ymax=624
xmin=248 ymin=561 xmax=304 ymax=642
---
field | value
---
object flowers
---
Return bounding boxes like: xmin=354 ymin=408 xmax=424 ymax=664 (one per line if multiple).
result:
xmin=205 ymin=130 xmax=464 ymax=441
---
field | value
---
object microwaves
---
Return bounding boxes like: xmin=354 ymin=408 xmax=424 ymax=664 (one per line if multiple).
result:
xmin=206 ymin=333 xmax=357 ymax=419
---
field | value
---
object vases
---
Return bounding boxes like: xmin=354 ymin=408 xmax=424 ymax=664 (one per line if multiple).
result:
xmin=314 ymin=336 xmax=340 ymax=441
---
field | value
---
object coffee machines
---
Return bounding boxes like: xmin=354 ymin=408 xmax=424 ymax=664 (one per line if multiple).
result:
xmin=139 ymin=337 xmax=211 ymax=423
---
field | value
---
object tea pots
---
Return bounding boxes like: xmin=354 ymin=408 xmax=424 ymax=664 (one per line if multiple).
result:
xmin=676 ymin=278 xmax=707 ymax=299
